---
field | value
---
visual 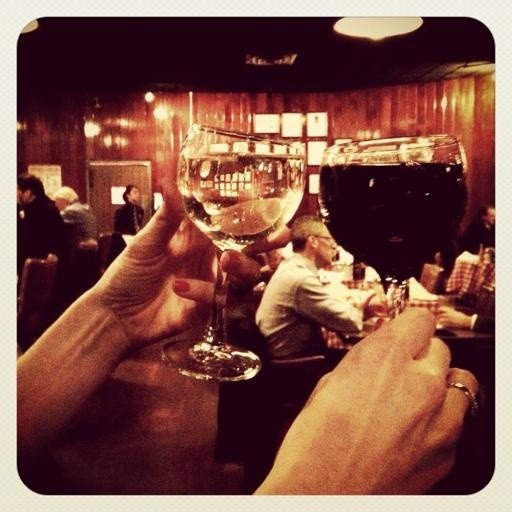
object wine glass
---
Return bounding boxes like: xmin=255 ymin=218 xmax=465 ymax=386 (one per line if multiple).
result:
xmin=160 ymin=124 xmax=307 ymax=383
xmin=318 ymin=133 xmax=471 ymax=326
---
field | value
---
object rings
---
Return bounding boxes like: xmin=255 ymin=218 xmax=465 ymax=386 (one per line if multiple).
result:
xmin=448 ymin=376 xmax=479 ymax=417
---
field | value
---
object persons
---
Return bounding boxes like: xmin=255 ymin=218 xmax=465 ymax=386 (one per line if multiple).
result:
xmin=51 ymin=183 xmax=102 ymax=271
xmin=254 ymin=215 xmax=362 ymax=372
xmin=17 ymin=177 xmax=483 ymax=493
xmin=18 ymin=172 xmax=66 ymax=296
xmin=460 ymin=206 xmax=494 ymax=254
xmin=108 ymin=183 xmax=145 ymax=266
xmin=432 ymin=299 xmax=493 ymax=344
